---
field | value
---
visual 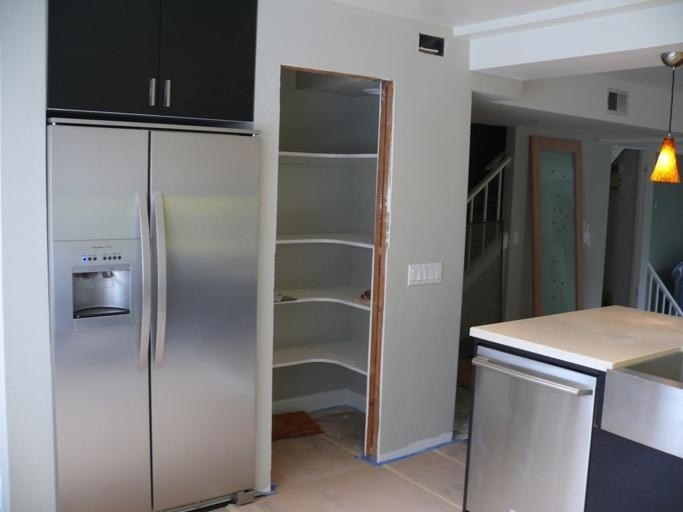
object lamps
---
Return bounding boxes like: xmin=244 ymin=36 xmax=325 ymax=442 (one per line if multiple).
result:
xmin=647 ymin=49 xmax=682 ymax=183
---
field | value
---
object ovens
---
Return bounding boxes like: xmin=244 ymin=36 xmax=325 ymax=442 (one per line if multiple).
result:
xmin=468 ymin=343 xmax=598 ymax=512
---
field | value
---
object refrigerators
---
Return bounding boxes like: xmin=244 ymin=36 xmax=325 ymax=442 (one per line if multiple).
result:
xmin=46 ymin=120 xmax=255 ymax=508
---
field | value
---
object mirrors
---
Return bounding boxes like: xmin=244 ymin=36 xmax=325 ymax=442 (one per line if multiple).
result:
xmin=529 ymin=132 xmax=585 ymax=318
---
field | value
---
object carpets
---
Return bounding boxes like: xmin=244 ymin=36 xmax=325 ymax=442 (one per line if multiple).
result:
xmin=272 ymin=409 xmax=327 ymax=441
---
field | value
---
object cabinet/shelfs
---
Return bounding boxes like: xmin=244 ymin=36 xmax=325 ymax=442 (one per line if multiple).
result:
xmin=45 ymin=0 xmax=257 ymax=123
xmin=270 ymin=148 xmax=379 ymax=377
xmin=460 ymin=305 xmax=683 ymax=511
